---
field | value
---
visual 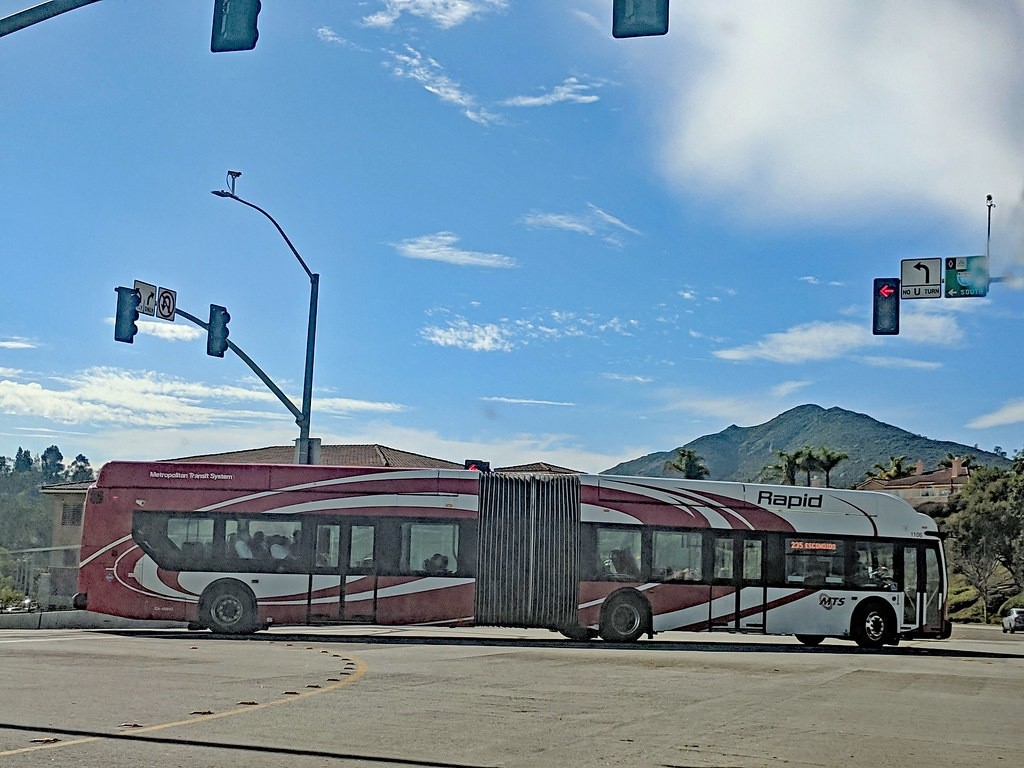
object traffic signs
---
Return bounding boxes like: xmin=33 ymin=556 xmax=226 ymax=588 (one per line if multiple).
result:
xmin=944 ymin=254 xmax=989 ymax=298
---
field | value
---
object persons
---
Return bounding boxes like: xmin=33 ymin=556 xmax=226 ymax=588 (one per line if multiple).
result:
xmin=600 ymin=548 xmax=684 ymax=580
xmin=794 ymin=549 xmax=893 ymax=589
xmin=428 ymin=553 xmax=453 ymax=577
xmin=235 ymin=528 xmax=307 ymax=571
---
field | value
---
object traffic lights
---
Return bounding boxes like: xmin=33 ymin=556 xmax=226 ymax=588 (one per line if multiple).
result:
xmin=464 ymin=458 xmax=491 ymax=474
xmin=871 ymin=276 xmax=900 ymax=337
xmin=113 ymin=286 xmax=142 ymax=344
xmin=205 ymin=303 xmax=231 ymax=358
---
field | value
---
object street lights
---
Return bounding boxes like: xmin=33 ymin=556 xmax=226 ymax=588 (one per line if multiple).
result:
xmin=211 ymin=168 xmax=323 ymax=464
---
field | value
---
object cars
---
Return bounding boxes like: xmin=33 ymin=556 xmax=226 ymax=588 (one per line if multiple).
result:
xmin=1001 ymin=608 xmax=1024 ymax=634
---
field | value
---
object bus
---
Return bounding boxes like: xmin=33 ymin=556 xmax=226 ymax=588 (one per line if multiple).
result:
xmin=68 ymin=458 xmax=957 ymax=649
